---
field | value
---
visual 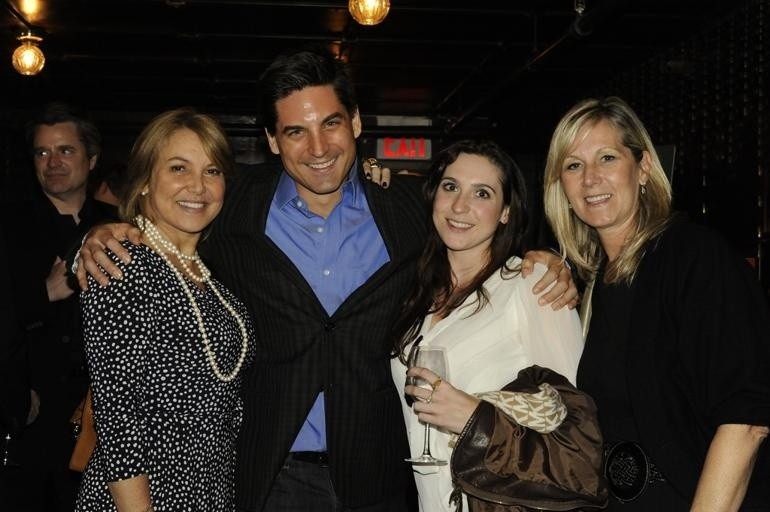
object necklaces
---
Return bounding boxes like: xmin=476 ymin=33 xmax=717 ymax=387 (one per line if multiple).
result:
xmin=131 ymin=214 xmax=250 ymax=381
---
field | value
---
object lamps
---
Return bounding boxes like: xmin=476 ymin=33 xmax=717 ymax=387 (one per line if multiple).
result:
xmin=347 ymin=0 xmax=391 ymax=26
xmin=0 ymin=0 xmax=47 ymax=76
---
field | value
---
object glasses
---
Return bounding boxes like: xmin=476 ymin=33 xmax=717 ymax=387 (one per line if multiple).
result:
xmin=403 ymin=335 xmax=423 ymax=408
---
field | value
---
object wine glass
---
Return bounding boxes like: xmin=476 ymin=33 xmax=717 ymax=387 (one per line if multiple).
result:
xmin=0 ymin=431 xmax=23 ymax=472
xmin=403 ymin=346 xmax=447 ymax=465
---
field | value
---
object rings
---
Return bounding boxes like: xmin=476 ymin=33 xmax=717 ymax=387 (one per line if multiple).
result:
xmin=367 ymin=156 xmax=383 ymax=168
xmin=425 ymin=392 xmax=435 ymax=403
xmin=430 ymin=377 xmax=443 ymax=389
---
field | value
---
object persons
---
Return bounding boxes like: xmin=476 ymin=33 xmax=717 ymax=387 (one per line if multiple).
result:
xmin=66 ymin=51 xmax=581 ymax=511
xmin=363 ymin=136 xmax=586 ymax=511
xmin=92 ymin=162 xmax=127 ymax=209
xmin=1 ymin=98 xmax=123 ymax=512
xmin=542 ymin=94 xmax=769 ymax=512
xmin=73 ymin=105 xmax=259 ymax=512
